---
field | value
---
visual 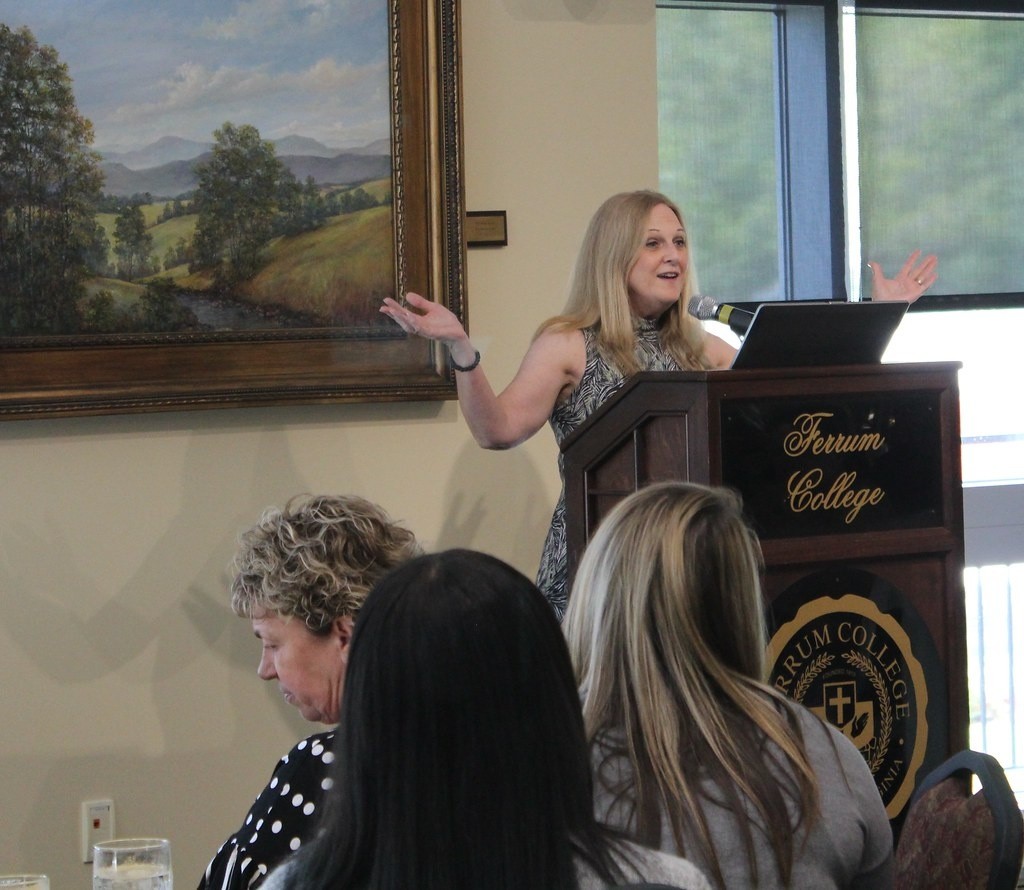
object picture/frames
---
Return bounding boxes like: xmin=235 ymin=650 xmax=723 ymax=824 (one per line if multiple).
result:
xmin=0 ymin=0 xmax=469 ymax=422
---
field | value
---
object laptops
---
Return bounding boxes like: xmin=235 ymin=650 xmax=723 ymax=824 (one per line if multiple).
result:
xmin=731 ymin=300 xmax=910 ymax=370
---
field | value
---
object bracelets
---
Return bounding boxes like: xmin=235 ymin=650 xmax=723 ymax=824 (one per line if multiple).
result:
xmin=449 ymin=351 xmax=480 ymax=371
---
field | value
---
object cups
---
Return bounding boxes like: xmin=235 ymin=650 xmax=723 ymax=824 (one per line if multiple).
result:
xmin=92 ymin=839 xmax=172 ymax=888
xmin=0 ymin=873 xmax=49 ymax=888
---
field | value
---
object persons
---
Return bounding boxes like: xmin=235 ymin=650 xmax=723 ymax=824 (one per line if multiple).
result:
xmin=378 ymin=190 xmax=937 ymax=619
xmin=191 ymin=481 xmax=896 ymax=890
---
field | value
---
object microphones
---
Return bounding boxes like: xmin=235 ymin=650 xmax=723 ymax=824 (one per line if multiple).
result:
xmin=688 ymin=297 xmax=755 ymax=335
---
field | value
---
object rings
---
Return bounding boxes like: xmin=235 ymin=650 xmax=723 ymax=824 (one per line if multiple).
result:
xmin=917 ymin=279 xmax=922 ymax=284
